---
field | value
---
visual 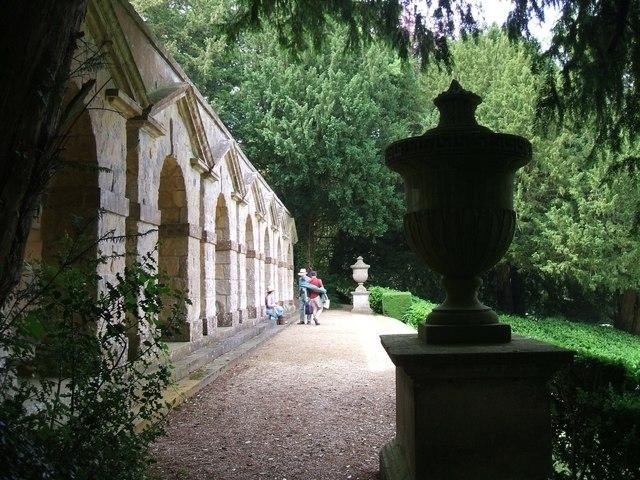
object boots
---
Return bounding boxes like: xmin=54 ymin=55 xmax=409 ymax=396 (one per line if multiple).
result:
xmin=277 ymin=315 xmax=288 ymax=325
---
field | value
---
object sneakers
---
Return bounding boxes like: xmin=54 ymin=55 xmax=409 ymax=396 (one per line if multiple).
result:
xmin=297 ymin=318 xmax=320 ymax=325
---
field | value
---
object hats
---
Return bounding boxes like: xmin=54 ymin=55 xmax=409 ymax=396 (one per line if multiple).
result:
xmin=298 ymin=269 xmax=308 ymax=275
xmin=267 ymin=285 xmax=275 ymax=293
xmin=309 ymin=271 xmax=316 ymax=276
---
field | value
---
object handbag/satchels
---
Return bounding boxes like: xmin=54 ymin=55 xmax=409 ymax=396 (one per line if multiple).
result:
xmin=323 ymin=294 xmax=330 ymax=309
xmin=305 ymin=300 xmax=313 ymax=314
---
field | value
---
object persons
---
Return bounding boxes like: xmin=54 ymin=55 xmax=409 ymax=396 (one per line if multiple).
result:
xmin=265 ymin=286 xmax=285 ymax=325
xmin=309 ymin=271 xmax=324 ymax=325
xmin=297 ymin=268 xmax=312 ymax=325
